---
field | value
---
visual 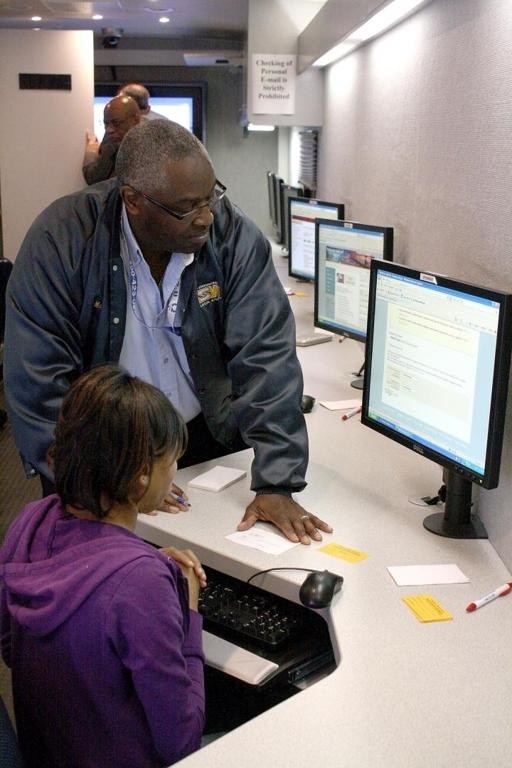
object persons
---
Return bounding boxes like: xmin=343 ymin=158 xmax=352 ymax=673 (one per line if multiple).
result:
xmin=103 ymin=95 xmax=141 ymax=179
xmin=83 ymin=83 xmax=152 ymax=185
xmin=3 ymin=118 xmax=334 ymax=545
xmin=0 ymin=361 xmax=206 ymax=768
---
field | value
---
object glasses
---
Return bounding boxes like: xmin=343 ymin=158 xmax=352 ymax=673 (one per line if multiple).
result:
xmin=124 ymin=177 xmax=227 ymax=221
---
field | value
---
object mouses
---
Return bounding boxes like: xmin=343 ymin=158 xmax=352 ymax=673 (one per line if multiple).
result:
xmin=301 ymin=395 xmax=316 ymax=413
xmin=299 ymin=568 xmax=344 ymax=609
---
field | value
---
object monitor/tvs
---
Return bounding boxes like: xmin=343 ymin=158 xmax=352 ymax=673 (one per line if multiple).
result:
xmin=267 ymin=172 xmax=304 ymax=251
xmin=359 ymin=257 xmax=512 ymax=539
xmin=313 ymin=216 xmax=395 ymax=390
xmin=288 ymin=195 xmax=345 ymax=282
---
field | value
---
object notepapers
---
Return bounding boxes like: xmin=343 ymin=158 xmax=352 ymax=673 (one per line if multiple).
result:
xmin=188 ymin=463 xmax=250 ymax=493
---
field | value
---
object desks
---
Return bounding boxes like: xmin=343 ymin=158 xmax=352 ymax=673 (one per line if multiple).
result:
xmin=134 ymin=234 xmax=510 ymax=767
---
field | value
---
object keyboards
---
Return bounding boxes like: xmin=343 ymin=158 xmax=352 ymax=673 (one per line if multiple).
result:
xmin=196 ymin=570 xmax=311 ymax=647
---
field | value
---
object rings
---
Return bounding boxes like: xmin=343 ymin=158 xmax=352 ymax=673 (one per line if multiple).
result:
xmin=301 ymin=515 xmax=309 ymax=520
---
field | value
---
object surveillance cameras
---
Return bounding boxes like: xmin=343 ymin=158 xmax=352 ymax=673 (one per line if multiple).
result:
xmin=101 ymin=26 xmax=123 ymax=49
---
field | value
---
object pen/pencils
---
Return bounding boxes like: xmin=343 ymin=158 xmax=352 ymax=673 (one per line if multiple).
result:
xmin=286 ymin=292 xmax=294 ymax=296
xmin=169 ymin=492 xmax=192 ymax=508
xmin=342 ymin=404 xmax=364 ymax=421
xmin=463 ymin=581 xmax=512 ymax=612
